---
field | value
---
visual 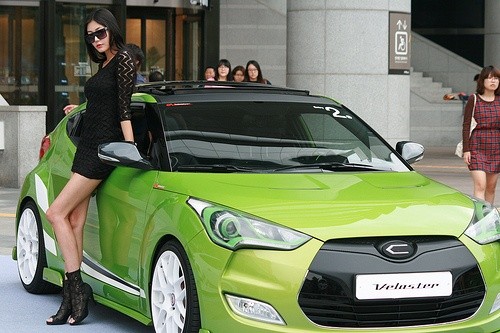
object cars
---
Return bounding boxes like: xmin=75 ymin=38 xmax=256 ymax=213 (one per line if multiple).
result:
xmin=12 ymin=80 xmax=499 ymax=333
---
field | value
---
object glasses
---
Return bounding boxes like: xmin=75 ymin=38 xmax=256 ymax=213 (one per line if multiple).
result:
xmin=86 ymin=27 xmax=107 ymax=44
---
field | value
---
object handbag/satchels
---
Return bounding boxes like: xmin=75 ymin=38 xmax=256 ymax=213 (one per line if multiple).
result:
xmin=455 ymin=93 xmax=477 ymax=158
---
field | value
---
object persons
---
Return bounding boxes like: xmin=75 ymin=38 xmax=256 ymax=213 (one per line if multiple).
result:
xmin=45 ymin=9 xmax=138 ymax=326
xmin=125 ymin=44 xmax=147 ymax=83
xmin=198 ymin=59 xmax=271 ymax=88
xmin=462 ymin=66 xmax=500 ymax=207
xmin=148 ymin=70 xmax=164 ymax=82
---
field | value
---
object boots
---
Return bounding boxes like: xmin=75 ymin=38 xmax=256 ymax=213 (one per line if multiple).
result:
xmin=47 ymin=280 xmax=71 ymax=325
xmin=65 ymin=268 xmax=96 ymax=325
